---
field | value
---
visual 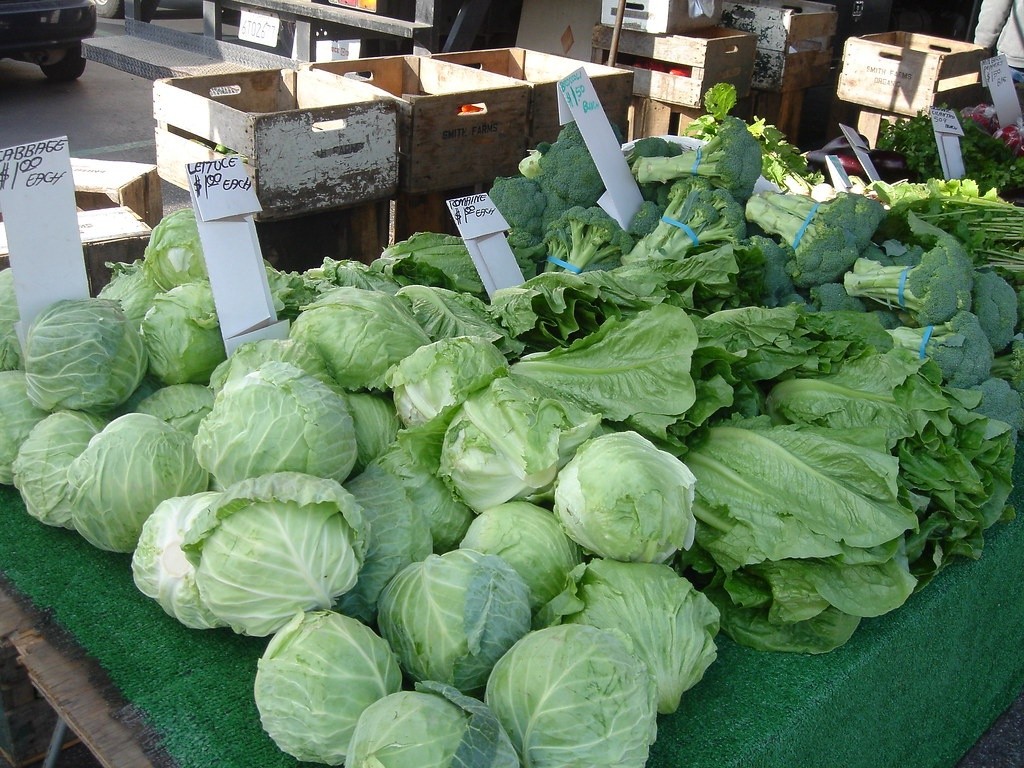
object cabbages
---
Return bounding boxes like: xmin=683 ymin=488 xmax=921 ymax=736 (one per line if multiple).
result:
xmin=1 ymin=212 xmax=720 ymax=768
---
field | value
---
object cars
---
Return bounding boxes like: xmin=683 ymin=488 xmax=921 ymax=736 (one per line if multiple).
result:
xmin=0 ymin=0 xmax=97 ymax=83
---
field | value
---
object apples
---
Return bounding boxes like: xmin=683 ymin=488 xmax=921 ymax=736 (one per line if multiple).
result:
xmin=631 ymin=56 xmax=691 ymax=78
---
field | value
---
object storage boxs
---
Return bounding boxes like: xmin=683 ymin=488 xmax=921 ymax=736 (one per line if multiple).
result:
xmin=430 ymin=47 xmax=634 ymax=155
xmin=312 ymin=54 xmax=529 ymax=195
xmin=70 ymin=157 xmax=163 ymax=228
xmin=836 ymin=30 xmax=987 ymax=118
xmin=152 ymin=68 xmax=399 ymax=224
xmin=0 ymin=206 xmax=153 ymax=297
xmin=590 ymin=0 xmax=838 ymax=147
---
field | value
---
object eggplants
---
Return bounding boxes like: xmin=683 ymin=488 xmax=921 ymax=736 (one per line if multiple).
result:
xmin=821 ymin=133 xmax=870 ymax=155
xmin=865 ymin=148 xmax=907 ymax=182
xmin=798 ymin=150 xmax=866 ymax=179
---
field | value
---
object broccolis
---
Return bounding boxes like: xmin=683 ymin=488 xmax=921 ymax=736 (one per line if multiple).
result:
xmin=485 ymin=115 xmax=1024 ymax=442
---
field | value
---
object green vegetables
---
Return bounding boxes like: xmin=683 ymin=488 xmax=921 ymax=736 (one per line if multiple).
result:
xmin=680 ymin=81 xmax=1024 ymax=274
xmin=302 ymin=233 xmax=1017 ymax=656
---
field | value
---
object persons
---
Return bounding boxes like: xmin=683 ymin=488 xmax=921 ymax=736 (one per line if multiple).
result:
xmin=973 ymin=0 xmax=1024 ymax=85
